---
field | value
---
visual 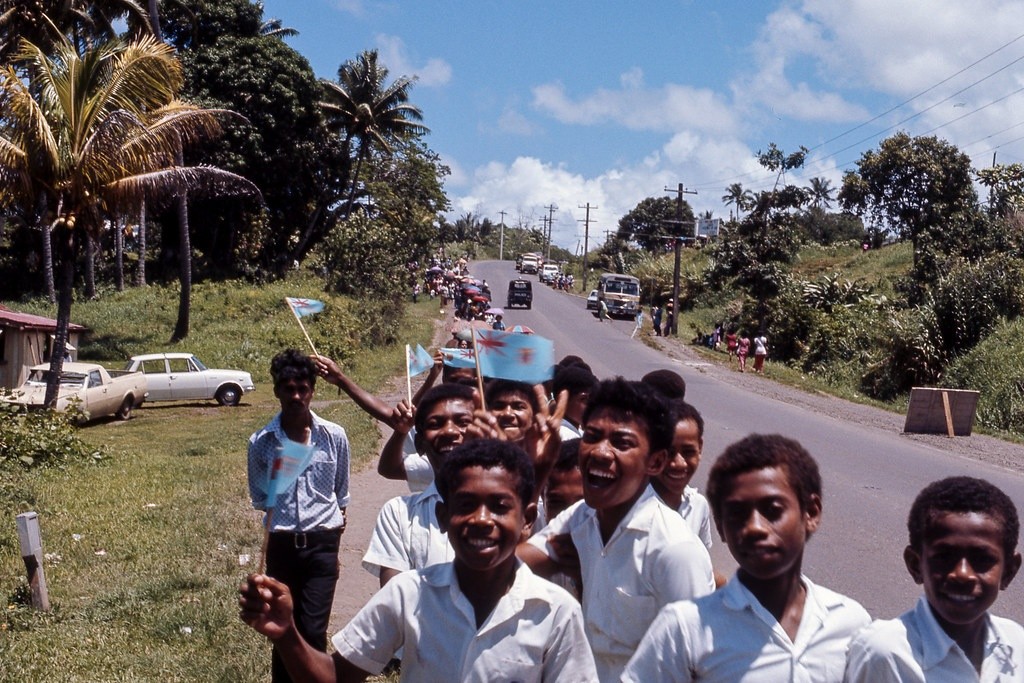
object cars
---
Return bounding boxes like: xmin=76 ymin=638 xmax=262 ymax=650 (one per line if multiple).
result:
xmin=586 ymin=289 xmax=598 ymax=309
xmin=122 ymin=353 xmax=254 ymax=408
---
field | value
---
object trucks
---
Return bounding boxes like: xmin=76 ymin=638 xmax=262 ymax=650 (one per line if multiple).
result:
xmin=515 ymin=251 xmax=543 ymax=274
xmin=538 ymin=265 xmax=558 ymax=283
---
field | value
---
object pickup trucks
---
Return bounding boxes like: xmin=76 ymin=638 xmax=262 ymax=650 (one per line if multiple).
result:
xmin=3 ymin=361 xmax=149 ymax=428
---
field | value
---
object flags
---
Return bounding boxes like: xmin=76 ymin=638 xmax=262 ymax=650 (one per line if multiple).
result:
xmin=264 ymin=437 xmax=315 ymax=508
xmin=287 ymin=297 xmax=324 ymax=319
xmin=440 ymin=347 xmax=477 ymax=369
xmin=406 ymin=343 xmax=434 ymax=378
xmin=473 ymin=329 xmax=554 ymax=385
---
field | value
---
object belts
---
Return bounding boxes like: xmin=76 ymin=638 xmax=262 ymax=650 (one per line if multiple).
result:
xmin=270 ymin=530 xmax=336 ymax=551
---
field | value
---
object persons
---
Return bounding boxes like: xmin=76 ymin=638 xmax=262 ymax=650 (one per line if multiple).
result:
xmin=597 ymin=296 xmax=613 ymax=323
xmin=621 ymin=432 xmax=873 ymax=683
xmin=238 ymin=252 xmax=1023 ymax=683
xmin=246 ymin=347 xmax=351 ymax=683
xmin=63 ymin=349 xmax=73 ymax=362
xmin=238 ymin=437 xmax=600 ymax=683
xmin=847 ymin=475 xmax=1023 ymax=683
xmin=753 ymin=330 xmax=768 ymax=373
xmin=738 ymin=330 xmax=751 ymax=373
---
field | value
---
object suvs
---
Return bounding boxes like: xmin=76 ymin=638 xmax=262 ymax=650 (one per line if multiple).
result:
xmin=507 ymin=279 xmax=532 ymax=309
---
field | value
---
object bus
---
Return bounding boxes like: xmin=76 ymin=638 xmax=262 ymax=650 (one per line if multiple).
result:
xmin=597 ymin=274 xmax=642 ymax=317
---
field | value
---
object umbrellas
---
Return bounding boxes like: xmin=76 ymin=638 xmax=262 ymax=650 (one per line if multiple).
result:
xmin=505 ymin=325 xmax=534 ymax=333
xmin=484 ymin=308 xmax=504 ymax=315
